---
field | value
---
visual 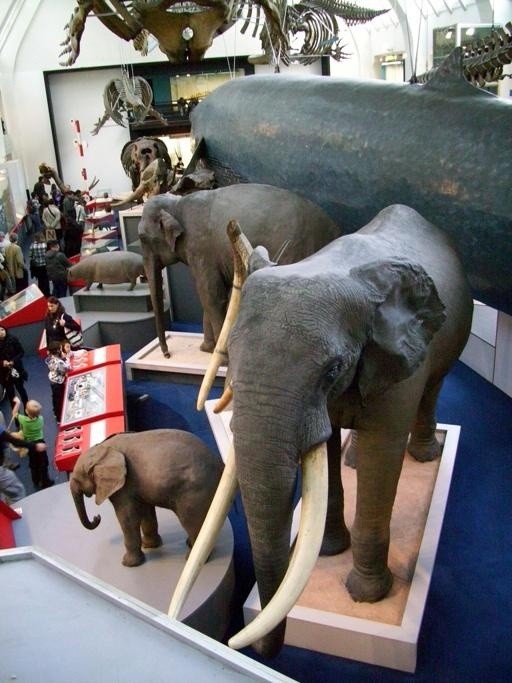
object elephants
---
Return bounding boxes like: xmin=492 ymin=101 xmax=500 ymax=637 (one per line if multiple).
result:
xmin=166 ymin=203 xmax=474 ymax=673
xmin=137 ymin=182 xmax=343 ymax=360
xmin=68 ymin=428 xmax=225 ymax=566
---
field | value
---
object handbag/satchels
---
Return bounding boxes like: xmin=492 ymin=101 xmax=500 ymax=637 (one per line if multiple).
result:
xmin=61 ymin=312 xmax=84 ymax=347
xmin=4 ymin=413 xmax=30 ymax=458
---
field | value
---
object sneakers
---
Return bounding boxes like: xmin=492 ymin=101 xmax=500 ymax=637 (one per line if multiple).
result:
xmin=5 ymin=462 xmax=55 ymax=488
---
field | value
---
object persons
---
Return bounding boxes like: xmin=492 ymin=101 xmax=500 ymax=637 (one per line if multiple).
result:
xmin=58 ymin=338 xmax=72 ymax=360
xmin=0 ymin=252 xmax=9 ymax=302
xmin=1 ymin=230 xmax=12 ymax=265
xmin=43 ymin=296 xmax=82 ymax=350
xmin=12 ymin=394 xmax=54 ymax=488
xmin=24 ymin=164 xmax=91 ymax=295
xmin=1 ymin=376 xmax=49 ymax=504
xmin=0 ymin=325 xmax=30 ymax=412
xmin=44 ymin=340 xmax=75 ymax=425
xmin=2 ymin=232 xmax=26 ymax=294
